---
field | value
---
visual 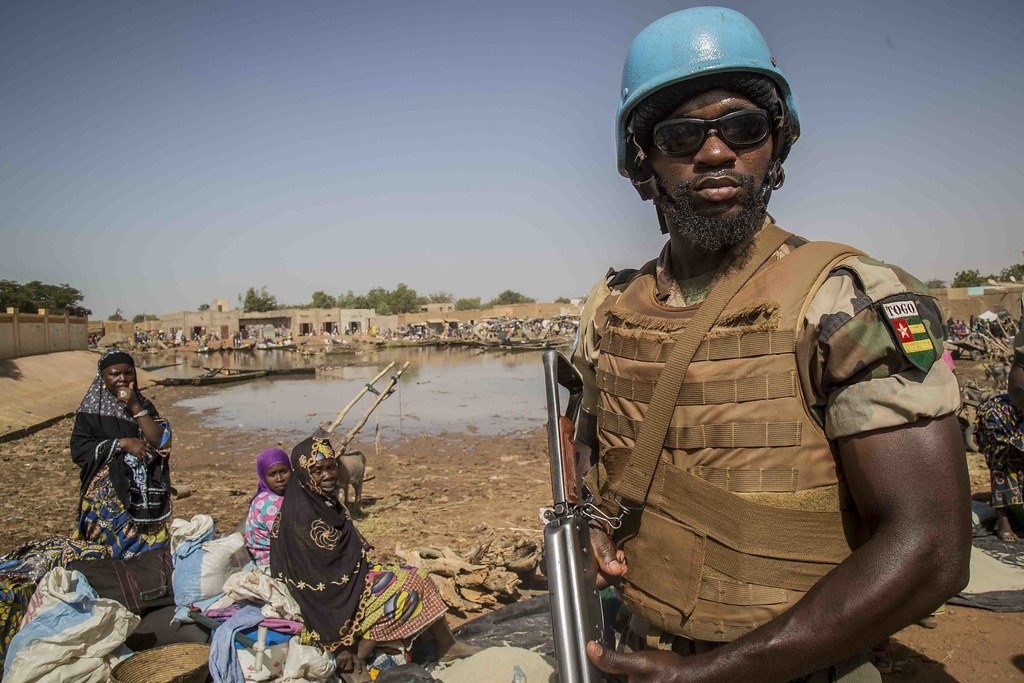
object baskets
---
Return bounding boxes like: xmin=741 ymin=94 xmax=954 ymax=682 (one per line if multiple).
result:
xmin=109 ymin=641 xmax=210 ymax=683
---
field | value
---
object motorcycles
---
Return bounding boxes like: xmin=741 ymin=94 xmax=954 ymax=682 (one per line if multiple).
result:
xmin=957 ymin=355 xmax=1011 ymax=452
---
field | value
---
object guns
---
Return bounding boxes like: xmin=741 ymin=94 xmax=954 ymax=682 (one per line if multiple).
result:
xmin=533 ymin=338 xmax=612 ymax=683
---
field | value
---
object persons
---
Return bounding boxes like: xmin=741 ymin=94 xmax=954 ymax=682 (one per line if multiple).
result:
xmin=88 ymin=323 xmax=360 ymax=344
xmin=567 ymin=7 xmax=973 ymax=683
xmin=367 ymin=316 xmax=583 ymax=340
xmin=70 ymin=347 xmax=173 ymax=560
xmin=269 ymin=436 xmax=484 ymax=683
xmin=947 ymin=310 xmax=1024 ymax=341
xmin=243 ymin=448 xmax=294 ymax=578
xmin=973 ymin=315 xmax=1024 ymax=541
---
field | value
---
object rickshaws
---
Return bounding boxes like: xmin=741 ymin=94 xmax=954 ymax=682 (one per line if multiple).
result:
xmin=947 ymin=332 xmax=992 ymax=360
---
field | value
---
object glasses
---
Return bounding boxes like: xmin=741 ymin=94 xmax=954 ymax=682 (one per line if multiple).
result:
xmin=649 ymin=105 xmax=779 ymax=158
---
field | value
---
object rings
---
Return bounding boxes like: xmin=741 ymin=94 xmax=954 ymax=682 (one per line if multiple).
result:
xmin=117 ymin=390 xmax=129 ymax=398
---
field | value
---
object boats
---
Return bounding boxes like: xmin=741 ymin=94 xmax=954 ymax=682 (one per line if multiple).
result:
xmin=379 ymin=318 xmax=578 ymax=350
xmin=194 ymin=339 xmax=355 ymax=354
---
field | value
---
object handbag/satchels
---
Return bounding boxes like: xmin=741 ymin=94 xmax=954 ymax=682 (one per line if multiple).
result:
xmin=66 ymin=546 xmax=174 ymax=614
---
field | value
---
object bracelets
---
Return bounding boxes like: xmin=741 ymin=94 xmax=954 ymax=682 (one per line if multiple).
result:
xmin=130 ymin=409 xmax=150 ymax=418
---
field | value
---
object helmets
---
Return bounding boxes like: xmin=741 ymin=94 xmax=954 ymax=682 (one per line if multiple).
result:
xmin=614 ymin=5 xmax=801 ymax=177
